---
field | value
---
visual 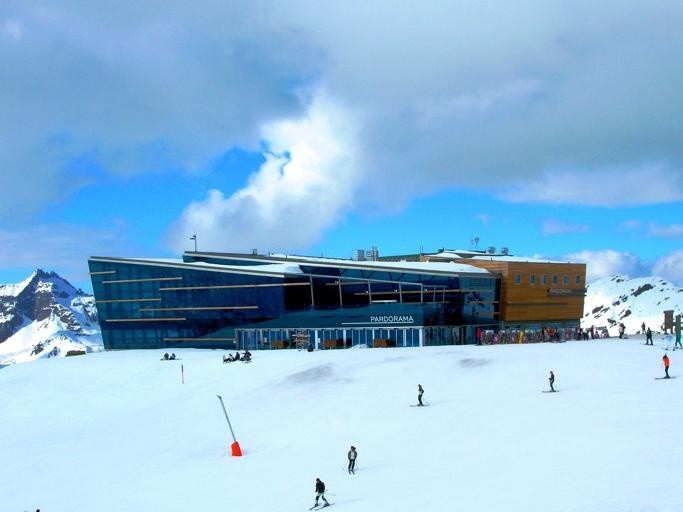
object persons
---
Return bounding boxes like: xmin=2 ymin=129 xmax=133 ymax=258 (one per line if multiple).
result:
xmin=314 ymin=478 xmax=330 ymax=507
xmin=662 ymin=353 xmax=670 ymax=378
xmin=476 ymin=325 xmax=609 ymax=345
xmin=223 ymin=349 xmax=252 ymax=364
xmin=348 ymin=446 xmax=357 ymax=475
xmin=417 ymin=384 xmax=424 ymax=406
xmin=619 ymin=322 xmax=653 ymax=345
xmin=549 ymin=371 xmax=555 ymax=391
xmin=160 ymin=352 xmax=175 ymax=360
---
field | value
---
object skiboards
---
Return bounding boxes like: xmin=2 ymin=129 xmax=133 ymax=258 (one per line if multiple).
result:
xmin=654 ymin=377 xmax=675 ymax=379
xmin=309 ymin=502 xmax=335 ymax=511
xmin=409 ymin=403 xmax=430 ymax=407
xmin=348 ymin=469 xmax=355 ymax=475
xmin=543 ymin=390 xmax=561 ymax=393
